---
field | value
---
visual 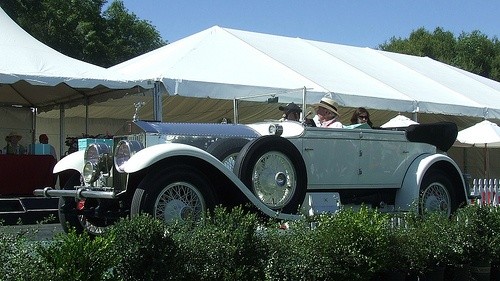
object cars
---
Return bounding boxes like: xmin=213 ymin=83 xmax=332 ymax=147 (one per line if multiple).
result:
xmin=31 ymin=86 xmax=482 ymax=245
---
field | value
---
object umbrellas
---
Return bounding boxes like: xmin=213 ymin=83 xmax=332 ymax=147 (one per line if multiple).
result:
xmin=452 ymin=119 xmax=500 ymax=182
xmin=380 ymin=111 xmax=420 ymax=128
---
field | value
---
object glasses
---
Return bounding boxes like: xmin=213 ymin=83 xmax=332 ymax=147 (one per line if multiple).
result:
xmin=357 ymin=114 xmax=369 ymax=120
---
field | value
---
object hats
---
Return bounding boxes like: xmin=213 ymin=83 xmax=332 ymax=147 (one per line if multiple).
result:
xmin=5 ymin=132 xmax=23 ymax=141
xmin=311 ymin=97 xmax=340 ymax=117
xmin=279 ymin=102 xmax=302 ymax=113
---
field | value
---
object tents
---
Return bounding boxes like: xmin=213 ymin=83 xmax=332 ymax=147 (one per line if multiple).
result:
xmin=107 ymin=25 xmax=500 ymax=142
xmin=0 ymin=0 xmax=110 ymax=160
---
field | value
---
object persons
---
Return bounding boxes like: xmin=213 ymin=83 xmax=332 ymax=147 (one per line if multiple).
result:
xmin=39 ymin=134 xmax=57 ymax=159
xmin=4 ymin=131 xmax=26 ymax=154
xmin=350 ymin=107 xmax=374 ymax=127
xmin=313 ymin=97 xmax=343 ymax=128
xmin=279 ymin=101 xmax=302 ymax=122
